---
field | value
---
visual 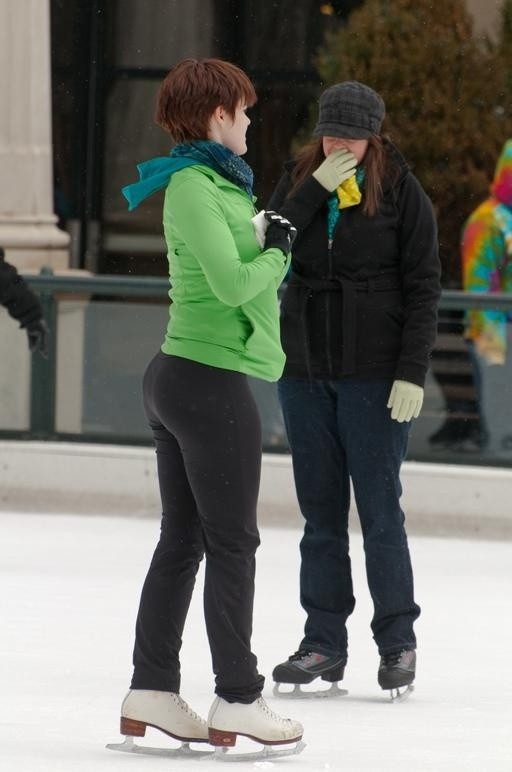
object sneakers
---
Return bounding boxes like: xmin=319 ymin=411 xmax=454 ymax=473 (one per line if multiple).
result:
xmin=207 ymin=698 xmax=302 ymax=747
xmin=378 ymin=648 xmax=416 ymax=690
xmin=274 ymin=650 xmax=347 ymax=684
xmin=120 ymin=689 xmax=209 ymax=742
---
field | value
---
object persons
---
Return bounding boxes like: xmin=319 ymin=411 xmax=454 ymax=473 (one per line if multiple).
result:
xmin=461 ymin=139 xmax=512 ymax=466
xmin=118 ymin=57 xmax=304 ymax=748
xmin=268 ymin=80 xmax=442 ymax=690
xmin=0 ymin=242 xmax=50 ymax=360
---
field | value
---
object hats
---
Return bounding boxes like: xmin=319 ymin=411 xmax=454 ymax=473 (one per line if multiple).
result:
xmin=313 ymin=80 xmax=386 ymax=139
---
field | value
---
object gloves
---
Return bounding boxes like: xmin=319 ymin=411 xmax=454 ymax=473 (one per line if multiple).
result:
xmin=387 ymin=381 xmax=424 ymax=423
xmin=26 ymin=319 xmax=52 ymax=361
xmin=262 ymin=209 xmax=296 ymax=257
xmin=313 ymin=149 xmax=358 ymax=191
